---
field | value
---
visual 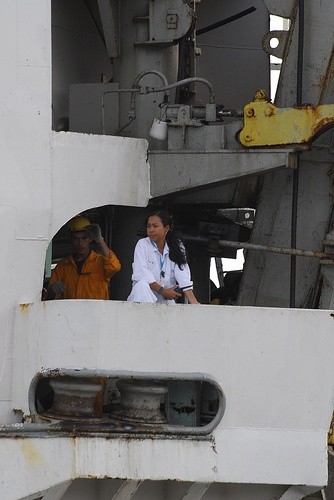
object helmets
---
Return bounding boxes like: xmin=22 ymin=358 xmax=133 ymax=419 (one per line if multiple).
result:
xmin=70 ymin=215 xmax=91 ymax=232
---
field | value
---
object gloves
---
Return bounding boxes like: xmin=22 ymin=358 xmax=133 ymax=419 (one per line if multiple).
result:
xmin=89 ymin=224 xmax=104 ymax=243
xmin=49 ymin=280 xmax=66 ymax=293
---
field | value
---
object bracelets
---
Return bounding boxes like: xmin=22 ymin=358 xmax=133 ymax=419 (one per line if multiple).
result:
xmin=158 ymin=286 xmax=163 ymax=295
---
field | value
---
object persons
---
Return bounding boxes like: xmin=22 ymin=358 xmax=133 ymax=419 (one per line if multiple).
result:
xmin=125 ymin=209 xmax=201 ymax=305
xmin=46 ymin=216 xmax=122 ymax=299
